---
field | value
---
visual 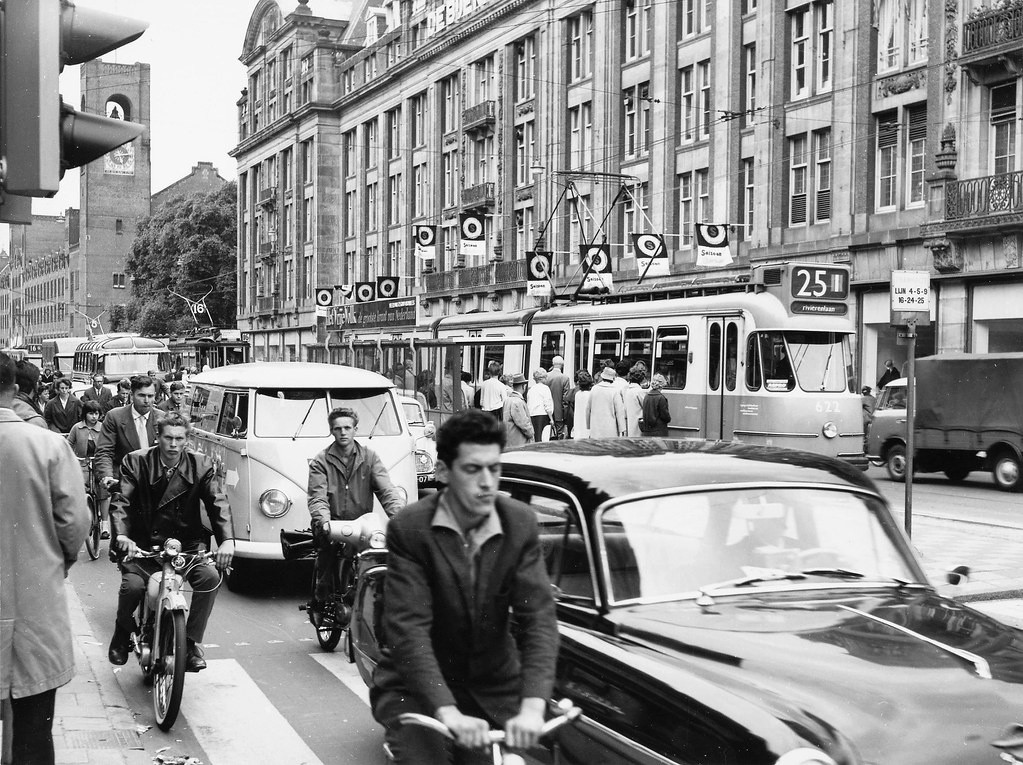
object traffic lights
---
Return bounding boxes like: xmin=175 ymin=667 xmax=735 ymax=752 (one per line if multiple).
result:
xmin=0 ymin=1 xmax=150 ymax=199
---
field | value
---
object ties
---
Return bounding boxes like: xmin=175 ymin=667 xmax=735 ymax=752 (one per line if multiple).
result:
xmin=137 ymin=415 xmax=150 ymax=450
xmin=122 ymin=403 xmax=124 ymax=406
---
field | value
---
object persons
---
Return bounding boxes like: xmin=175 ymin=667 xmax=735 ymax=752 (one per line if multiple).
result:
xmin=775 ymin=345 xmax=792 ymax=378
xmin=307 ymin=408 xmax=406 ymax=612
xmin=108 ymin=413 xmax=235 ymax=670
xmin=12 ymin=358 xmax=198 ymax=540
xmin=368 ymin=407 xmax=558 ymax=765
xmin=877 ymin=359 xmax=901 ymax=391
xmin=0 ymin=350 xmax=93 ymax=765
xmin=373 ymin=356 xmax=671 ymax=448
xmin=860 ymin=386 xmax=877 ymax=423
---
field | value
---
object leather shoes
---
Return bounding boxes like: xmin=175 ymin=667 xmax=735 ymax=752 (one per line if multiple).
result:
xmin=108 ymin=634 xmax=128 ymax=665
xmin=185 ymin=645 xmax=206 ymax=669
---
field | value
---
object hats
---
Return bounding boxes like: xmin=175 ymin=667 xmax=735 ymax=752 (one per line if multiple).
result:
xmin=601 ymin=367 xmax=616 ymax=381
xmin=508 ymin=373 xmax=529 ymax=383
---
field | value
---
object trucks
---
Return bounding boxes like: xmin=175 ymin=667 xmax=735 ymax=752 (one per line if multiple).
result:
xmin=865 ymin=352 xmax=1022 ymax=493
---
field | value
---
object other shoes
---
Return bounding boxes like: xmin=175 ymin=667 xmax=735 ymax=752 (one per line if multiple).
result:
xmin=101 ymin=526 xmax=109 ymax=537
xmin=309 ymin=604 xmax=323 ymax=627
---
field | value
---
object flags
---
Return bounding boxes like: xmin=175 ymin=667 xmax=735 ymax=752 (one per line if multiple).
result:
xmin=414 ymin=224 xmax=436 ymax=261
xmin=630 ymin=233 xmax=671 ymax=277
xmin=578 ymin=244 xmax=615 ymax=292
xmin=377 ymin=276 xmax=400 ymax=299
xmin=524 ymin=251 xmax=553 ymax=297
xmin=356 ymin=281 xmax=377 ymax=302
xmin=694 ymin=222 xmax=734 ymax=267
xmin=458 ymin=212 xmax=487 ymax=255
xmin=333 ymin=284 xmax=354 ymax=300
xmin=315 ymin=287 xmax=334 ymax=307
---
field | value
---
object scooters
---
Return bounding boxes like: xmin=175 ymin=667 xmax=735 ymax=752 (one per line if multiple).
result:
xmin=299 ymin=510 xmax=391 ymax=661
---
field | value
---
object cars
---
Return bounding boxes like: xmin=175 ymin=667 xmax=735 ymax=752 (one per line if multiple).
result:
xmin=497 ymin=437 xmax=1023 ymax=765
xmin=398 ymin=394 xmax=439 ymax=485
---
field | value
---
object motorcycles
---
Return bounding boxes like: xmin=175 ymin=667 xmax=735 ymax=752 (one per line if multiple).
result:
xmin=115 ymin=537 xmax=235 ymax=733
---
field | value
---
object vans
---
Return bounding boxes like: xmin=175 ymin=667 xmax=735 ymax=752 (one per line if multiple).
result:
xmin=181 ymin=361 xmax=436 ymax=598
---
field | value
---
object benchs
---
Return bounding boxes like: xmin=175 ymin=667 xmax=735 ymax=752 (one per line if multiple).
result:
xmin=537 ymin=535 xmax=678 ymax=603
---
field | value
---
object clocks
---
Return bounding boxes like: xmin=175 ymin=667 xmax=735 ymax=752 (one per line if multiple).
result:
xmin=105 ymin=144 xmax=134 ymax=170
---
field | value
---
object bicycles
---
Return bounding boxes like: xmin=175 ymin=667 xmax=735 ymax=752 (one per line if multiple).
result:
xmin=78 ymin=456 xmax=103 ymax=561
xmin=382 ymin=694 xmax=585 ymax=765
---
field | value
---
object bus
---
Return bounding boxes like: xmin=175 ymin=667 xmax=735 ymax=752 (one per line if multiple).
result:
xmin=429 ymin=170 xmax=870 ymax=472
xmin=0 ymin=303 xmax=173 ymax=387
